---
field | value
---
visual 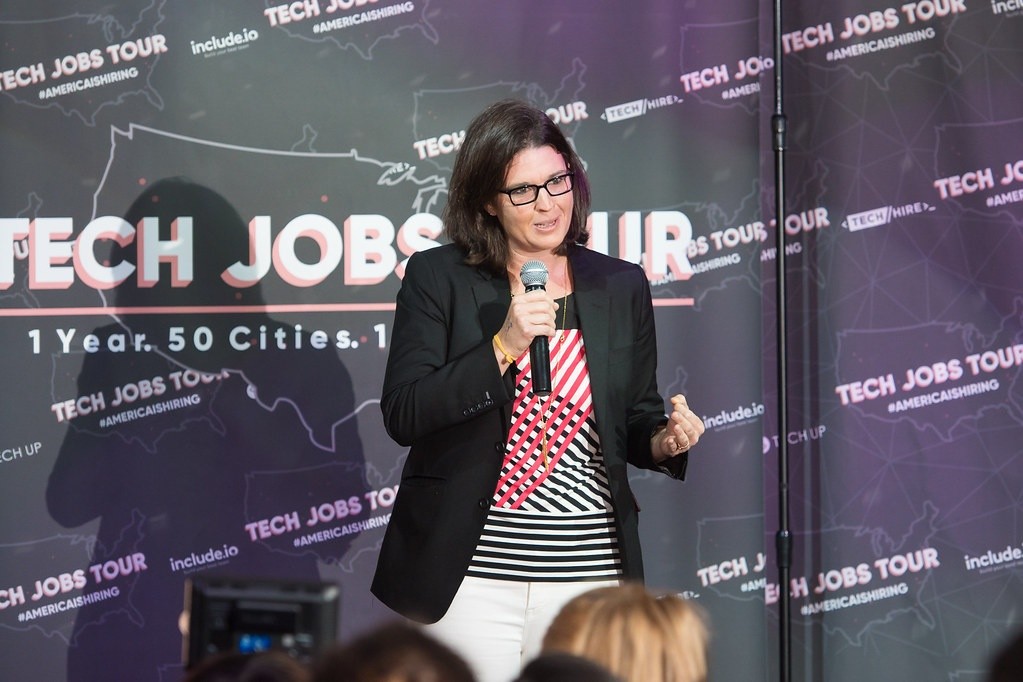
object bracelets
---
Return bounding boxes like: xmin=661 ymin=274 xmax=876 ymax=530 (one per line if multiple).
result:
xmin=494 ymin=334 xmax=518 ymax=363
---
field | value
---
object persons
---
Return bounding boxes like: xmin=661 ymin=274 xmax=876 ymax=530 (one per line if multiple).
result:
xmin=182 ymin=585 xmax=711 ymax=681
xmin=369 ymin=97 xmax=705 ymax=682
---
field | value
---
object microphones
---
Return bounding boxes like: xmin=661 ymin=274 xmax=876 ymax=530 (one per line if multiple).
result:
xmin=520 ymin=260 xmax=551 ymax=397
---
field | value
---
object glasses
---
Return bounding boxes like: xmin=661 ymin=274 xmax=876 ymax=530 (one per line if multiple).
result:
xmin=499 ymin=169 xmax=574 ymax=206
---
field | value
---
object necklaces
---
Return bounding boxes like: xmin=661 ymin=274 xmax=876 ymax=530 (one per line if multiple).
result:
xmin=510 ymin=261 xmax=567 ymax=475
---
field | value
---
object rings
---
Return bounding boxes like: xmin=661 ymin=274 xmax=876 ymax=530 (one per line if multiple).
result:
xmin=677 ymin=443 xmax=690 ymax=450
